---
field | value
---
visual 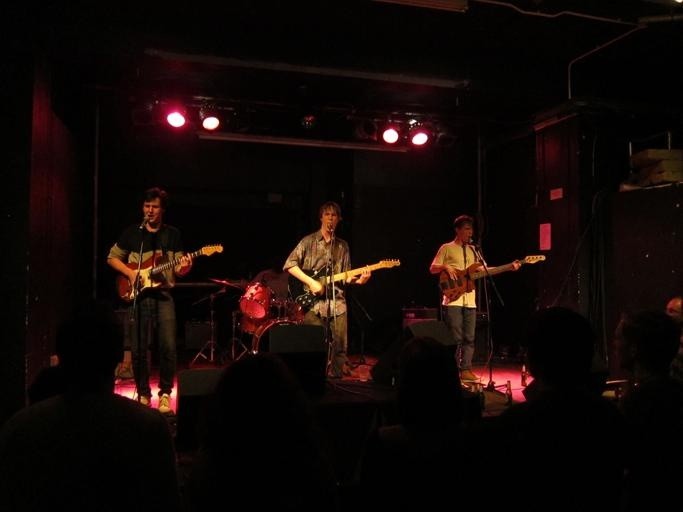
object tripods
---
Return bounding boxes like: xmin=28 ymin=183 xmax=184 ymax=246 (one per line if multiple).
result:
xmin=463 ymin=253 xmax=508 ymax=399
xmin=342 ymin=288 xmax=376 ymax=371
xmin=188 ymin=301 xmax=230 ymax=368
xmin=324 ymin=236 xmax=373 ymax=394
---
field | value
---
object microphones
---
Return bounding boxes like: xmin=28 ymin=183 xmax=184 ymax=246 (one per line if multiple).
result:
xmin=326 ymin=222 xmax=334 ymax=233
xmin=468 ymin=239 xmax=480 ymax=252
xmin=214 ymin=287 xmax=226 ymax=295
xmin=139 ymin=215 xmax=149 ymax=229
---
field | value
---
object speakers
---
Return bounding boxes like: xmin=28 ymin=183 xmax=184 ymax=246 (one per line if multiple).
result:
xmin=176 ymin=370 xmax=222 ymax=432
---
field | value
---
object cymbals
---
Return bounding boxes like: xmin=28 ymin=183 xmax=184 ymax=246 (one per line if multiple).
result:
xmin=210 ymin=278 xmax=240 ymax=289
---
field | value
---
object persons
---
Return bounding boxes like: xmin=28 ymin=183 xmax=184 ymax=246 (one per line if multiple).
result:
xmin=429 ymin=213 xmax=522 ymax=380
xmin=109 ymin=186 xmax=194 ymax=415
xmin=0 ymin=298 xmax=683 ymax=511
xmin=281 ymin=202 xmax=373 ymax=384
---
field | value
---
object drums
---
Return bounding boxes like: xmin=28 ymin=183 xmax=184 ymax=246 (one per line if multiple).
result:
xmin=271 ymin=299 xmax=305 ymax=321
xmin=252 ymin=318 xmax=304 ymax=354
xmin=240 ymin=282 xmax=273 ymax=321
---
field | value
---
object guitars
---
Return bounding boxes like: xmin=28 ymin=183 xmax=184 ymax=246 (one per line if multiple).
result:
xmin=288 ymin=259 xmax=401 ymax=312
xmin=114 ymin=244 xmax=225 ymax=306
xmin=440 ymin=255 xmax=546 ymax=300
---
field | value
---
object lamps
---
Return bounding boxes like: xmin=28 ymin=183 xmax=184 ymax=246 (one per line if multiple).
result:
xmin=152 ymin=99 xmax=233 ymax=133
xmin=372 ymin=113 xmax=436 ymax=148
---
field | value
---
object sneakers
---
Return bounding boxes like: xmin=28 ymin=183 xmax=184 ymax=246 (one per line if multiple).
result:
xmin=158 ymin=393 xmax=170 ymax=413
xmin=135 ymin=394 xmax=151 ymax=406
xmin=462 ymin=369 xmax=480 ymax=380
